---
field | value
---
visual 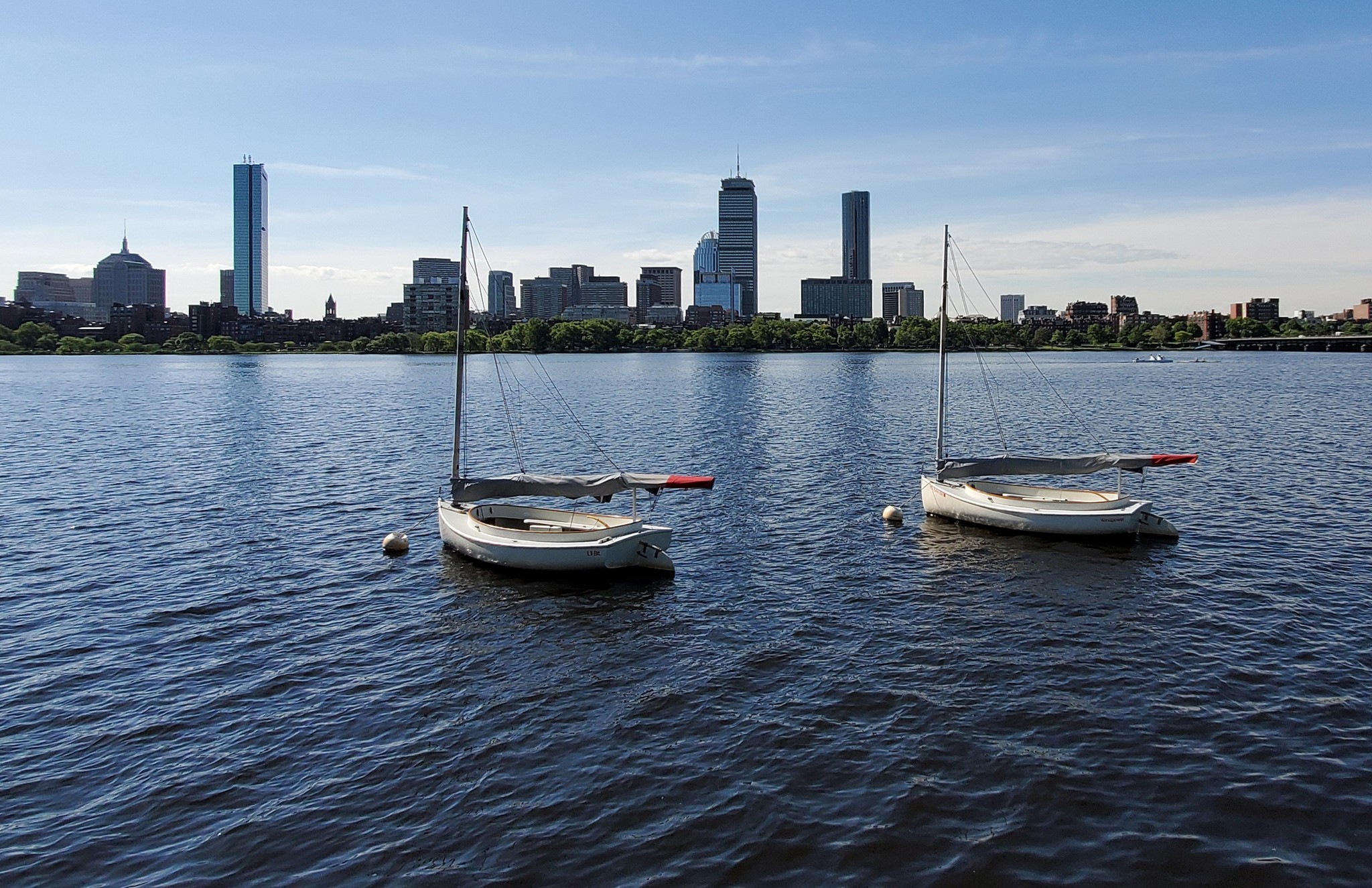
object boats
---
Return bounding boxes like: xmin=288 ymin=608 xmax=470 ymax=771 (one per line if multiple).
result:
xmin=1132 ymin=354 xmax=1175 ymax=363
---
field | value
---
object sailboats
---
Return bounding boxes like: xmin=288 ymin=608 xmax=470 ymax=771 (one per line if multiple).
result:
xmin=919 ymin=224 xmax=1202 ymax=538
xmin=436 ymin=205 xmax=718 ymax=571
